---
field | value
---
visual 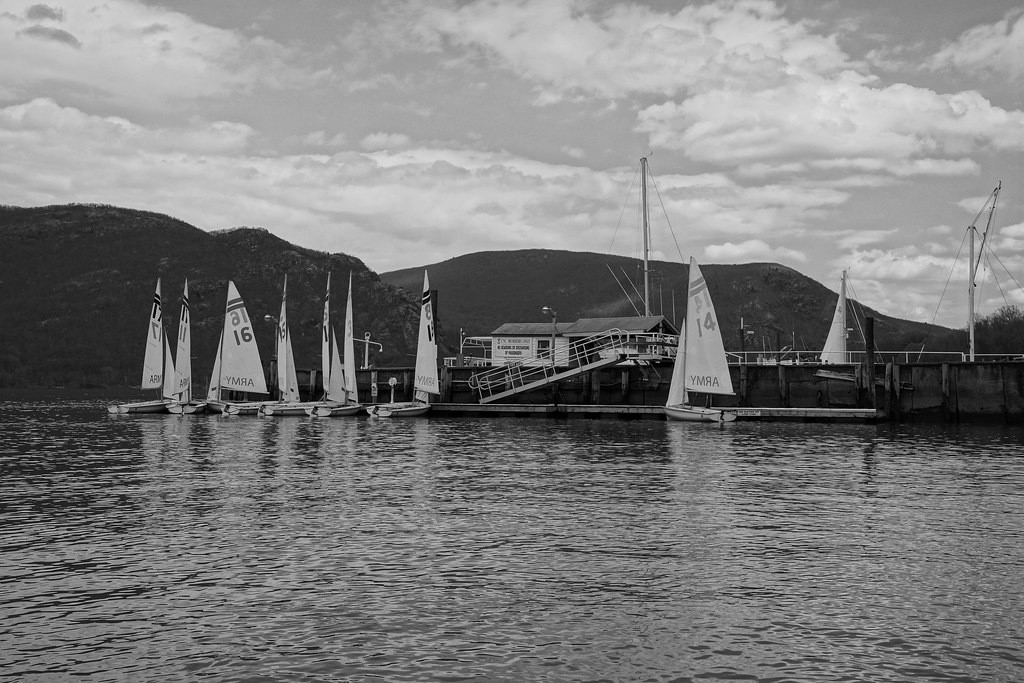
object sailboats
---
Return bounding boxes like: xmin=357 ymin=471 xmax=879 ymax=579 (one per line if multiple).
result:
xmin=257 ymin=270 xmax=344 ymax=417
xmin=429 ymin=156 xmax=1024 ymax=417
xmin=364 ymin=270 xmax=441 ymax=417
xmin=223 ymin=273 xmax=301 ymax=413
xmin=106 ymin=278 xmax=173 ymax=414
xmin=305 ymin=271 xmax=371 ymax=418
xmin=665 ymin=253 xmax=738 ymax=423
xmin=165 ymin=278 xmax=220 ymax=414
xmin=205 ymin=280 xmax=271 ymax=412
